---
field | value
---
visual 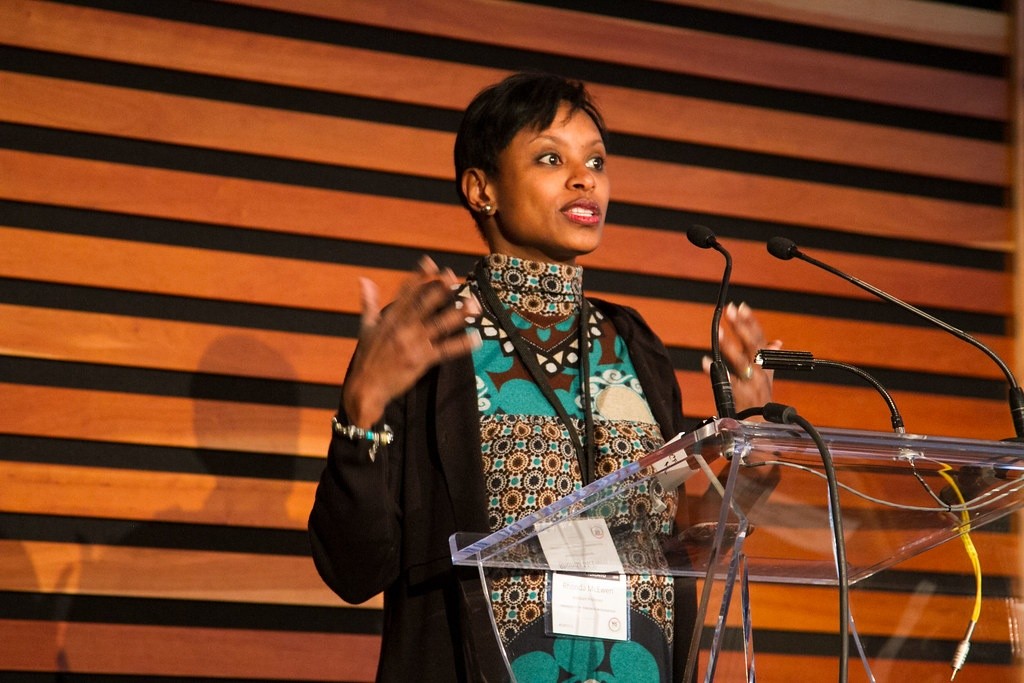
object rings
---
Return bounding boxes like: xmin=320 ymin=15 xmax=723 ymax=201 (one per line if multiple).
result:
xmin=739 ymin=359 xmax=755 ymax=381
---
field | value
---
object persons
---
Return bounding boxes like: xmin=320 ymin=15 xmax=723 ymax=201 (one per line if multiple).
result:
xmin=305 ymin=70 xmax=785 ymax=682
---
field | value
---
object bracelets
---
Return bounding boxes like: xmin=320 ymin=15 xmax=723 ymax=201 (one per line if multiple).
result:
xmin=331 ymin=411 xmax=394 ymax=447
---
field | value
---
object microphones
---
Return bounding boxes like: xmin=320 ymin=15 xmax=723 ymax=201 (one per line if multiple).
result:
xmin=766 ymin=236 xmax=1024 ymax=444
xmin=686 ymin=224 xmax=737 ymax=421
